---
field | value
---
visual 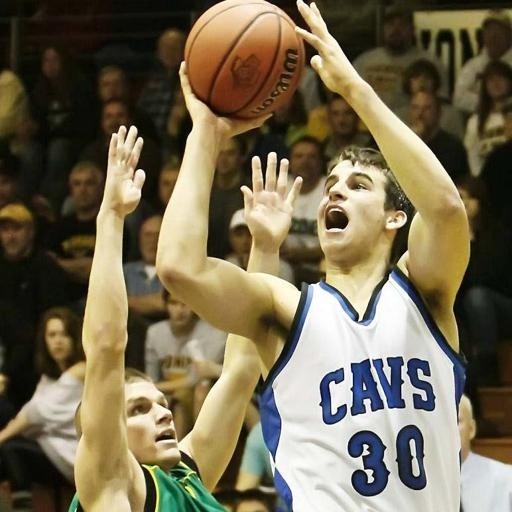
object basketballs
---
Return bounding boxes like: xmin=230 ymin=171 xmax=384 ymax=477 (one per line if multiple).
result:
xmin=184 ymin=0 xmax=306 ymax=119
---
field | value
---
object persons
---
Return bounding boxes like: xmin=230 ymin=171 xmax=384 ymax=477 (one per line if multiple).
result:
xmin=0 ymin=0 xmax=512 ymax=512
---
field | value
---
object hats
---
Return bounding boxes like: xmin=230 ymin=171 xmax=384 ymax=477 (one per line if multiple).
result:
xmin=0 ymin=206 xmax=31 ymax=222
xmin=230 ymin=209 xmax=248 ymax=229
